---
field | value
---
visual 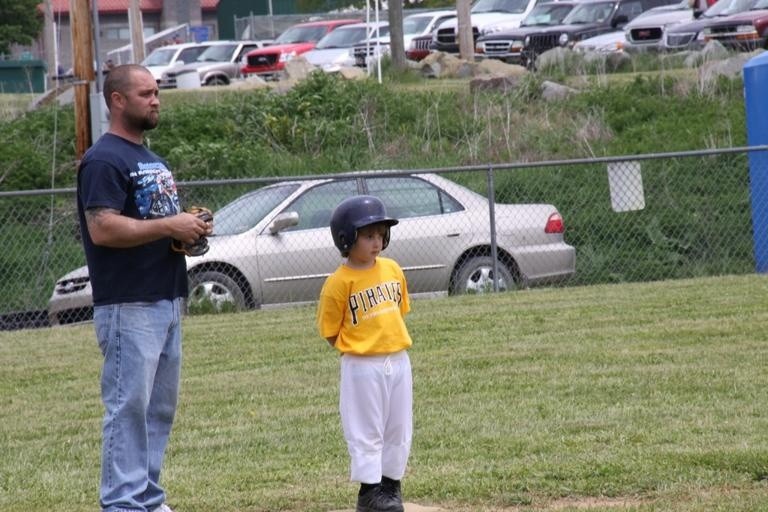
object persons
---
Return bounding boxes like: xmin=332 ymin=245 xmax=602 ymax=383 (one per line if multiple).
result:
xmin=316 ymin=195 xmax=415 ymax=512
xmin=76 ymin=62 xmax=215 ymax=512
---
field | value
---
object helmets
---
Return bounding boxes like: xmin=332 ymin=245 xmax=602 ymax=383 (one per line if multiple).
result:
xmin=330 ymin=195 xmax=399 ymax=257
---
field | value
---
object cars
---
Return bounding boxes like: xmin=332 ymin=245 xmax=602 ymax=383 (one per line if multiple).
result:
xmin=46 ymin=169 xmax=577 ymax=326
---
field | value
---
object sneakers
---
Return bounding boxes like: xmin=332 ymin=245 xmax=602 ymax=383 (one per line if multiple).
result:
xmin=356 ymin=477 xmax=405 ymax=511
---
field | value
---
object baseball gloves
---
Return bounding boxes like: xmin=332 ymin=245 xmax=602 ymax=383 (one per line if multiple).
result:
xmin=169 ymin=205 xmax=214 ymax=257
